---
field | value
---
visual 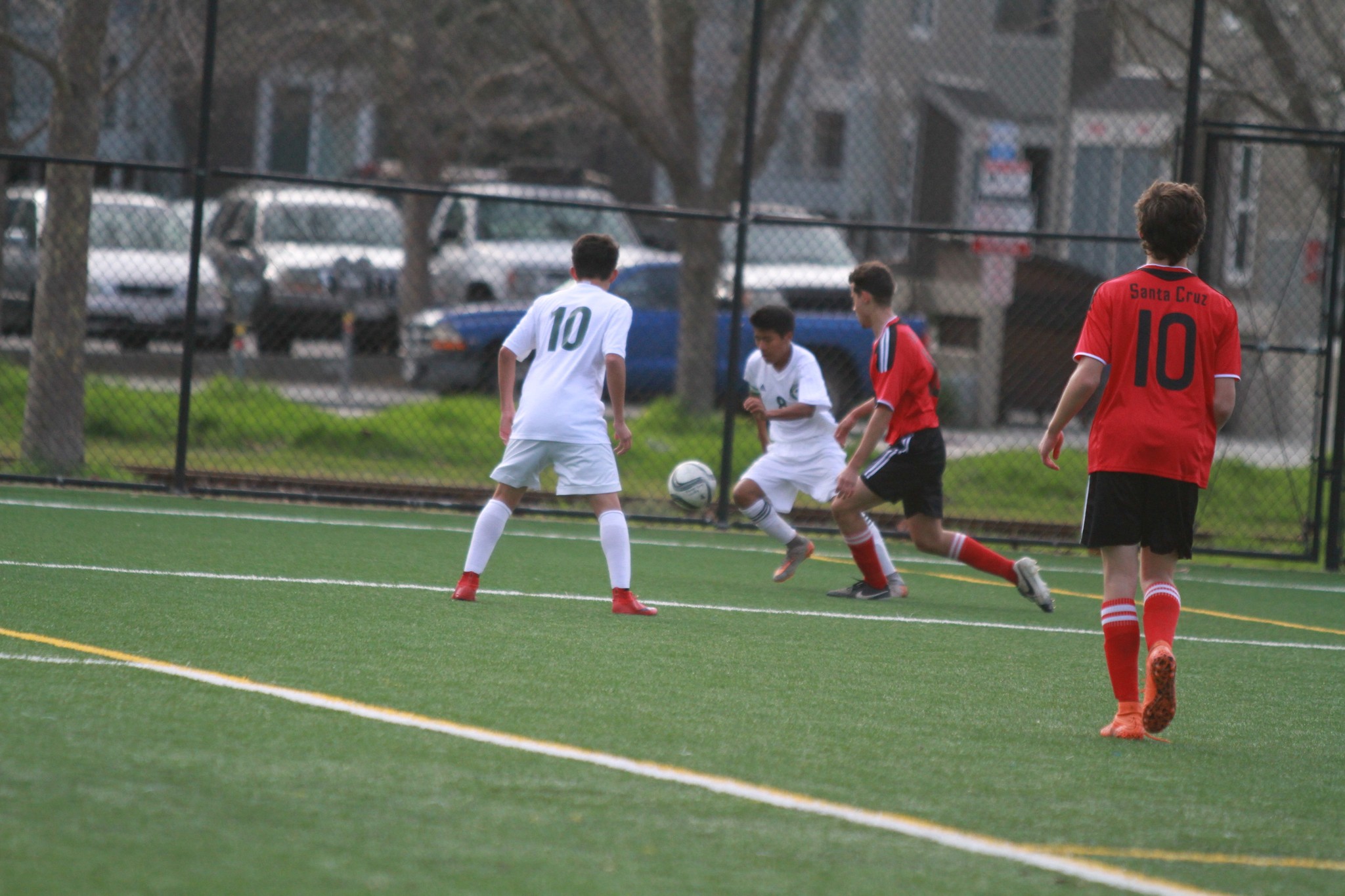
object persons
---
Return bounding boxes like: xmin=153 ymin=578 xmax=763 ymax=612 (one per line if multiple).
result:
xmin=829 ymin=262 xmax=1056 ymax=614
xmin=1039 ymin=180 xmax=1241 ymax=738
xmin=452 ymin=232 xmax=662 ymax=615
xmin=731 ymin=304 xmax=909 ymax=601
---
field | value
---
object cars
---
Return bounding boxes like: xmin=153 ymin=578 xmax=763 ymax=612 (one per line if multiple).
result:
xmin=0 ymin=183 xmax=230 ymax=356
xmin=425 ymin=178 xmax=682 ymax=312
xmin=398 ymin=251 xmax=933 ymax=417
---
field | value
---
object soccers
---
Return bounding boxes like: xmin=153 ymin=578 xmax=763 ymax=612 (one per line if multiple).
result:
xmin=667 ymin=460 xmax=717 ymax=510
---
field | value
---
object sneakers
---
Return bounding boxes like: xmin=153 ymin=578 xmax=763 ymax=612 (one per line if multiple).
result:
xmin=450 ymin=581 xmax=476 ymax=601
xmin=827 ymin=580 xmax=891 ymax=601
xmin=1013 ymin=557 xmax=1056 ymax=613
xmin=773 ymin=539 xmax=814 ymax=582
xmin=888 ymin=572 xmax=907 ymax=597
xmin=1139 ymin=646 xmax=1177 ymax=734
xmin=1099 ymin=712 xmax=1146 ymax=741
xmin=611 ymin=590 xmax=657 ymax=616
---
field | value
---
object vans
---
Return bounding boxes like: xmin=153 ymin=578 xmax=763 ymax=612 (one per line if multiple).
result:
xmin=199 ymin=178 xmax=404 ymax=360
xmin=714 ymin=198 xmax=862 ymax=311
